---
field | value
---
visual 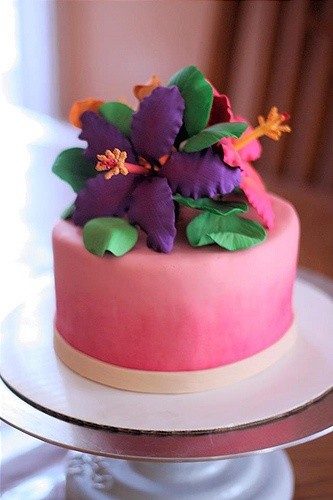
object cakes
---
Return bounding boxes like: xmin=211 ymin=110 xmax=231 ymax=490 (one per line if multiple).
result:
xmin=50 ymin=63 xmax=300 ymax=394
